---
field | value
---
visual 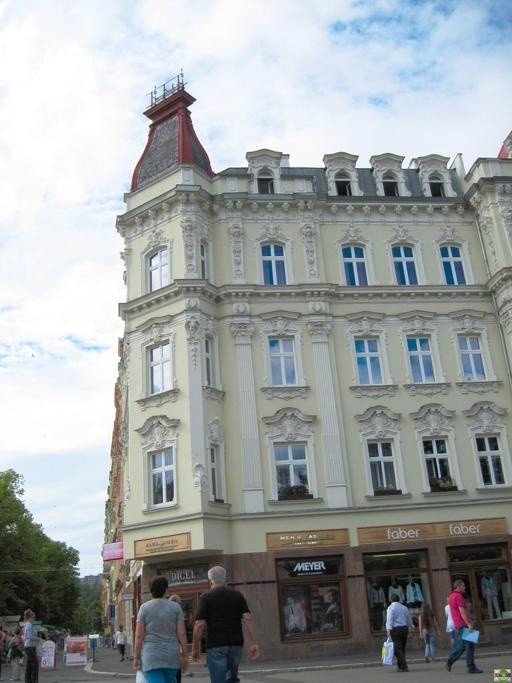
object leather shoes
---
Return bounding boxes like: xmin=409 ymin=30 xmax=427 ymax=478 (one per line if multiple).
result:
xmin=398 ymin=668 xmax=409 ymax=672
xmin=468 ymin=668 xmax=483 ymax=673
xmin=444 ymin=664 xmax=451 ymax=672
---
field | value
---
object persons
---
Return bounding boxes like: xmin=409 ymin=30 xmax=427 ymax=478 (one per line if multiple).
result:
xmin=385 ymin=593 xmax=416 ymax=673
xmin=388 ymin=581 xmax=406 ymax=604
xmin=131 ymin=575 xmax=190 ymax=682
xmin=370 ymin=582 xmax=387 ymax=611
xmin=284 ymin=594 xmax=307 ymax=631
xmin=192 ymin=564 xmax=260 ymax=682
xmin=444 ymin=593 xmax=458 ymax=651
xmin=325 ymin=588 xmax=342 ymax=629
xmin=444 ymin=579 xmax=484 ymax=672
xmin=113 ymin=624 xmax=127 ymax=661
xmin=419 ymin=603 xmax=442 ymax=662
xmin=406 ymin=578 xmax=425 ymax=608
xmin=0 ymin=606 xmax=71 ymax=682
xmin=481 ymin=574 xmax=502 ymax=618
xmin=168 ymin=594 xmax=195 ymax=677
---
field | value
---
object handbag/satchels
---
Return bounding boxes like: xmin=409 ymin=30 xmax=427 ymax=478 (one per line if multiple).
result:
xmin=381 ymin=636 xmax=393 ymax=665
